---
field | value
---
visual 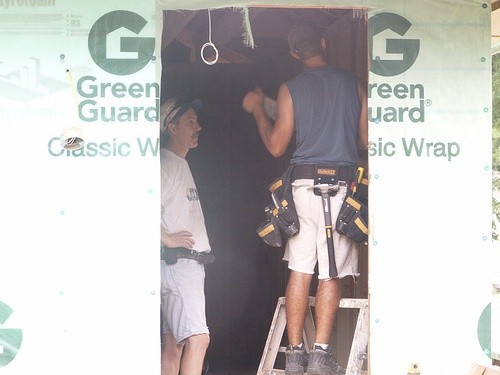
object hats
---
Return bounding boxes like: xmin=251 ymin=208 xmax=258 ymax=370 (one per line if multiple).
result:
xmin=160 ymin=96 xmax=184 ymax=146
xmin=287 ymin=21 xmax=323 ymax=56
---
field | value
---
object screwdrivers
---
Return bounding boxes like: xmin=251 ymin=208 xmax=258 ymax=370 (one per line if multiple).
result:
xmin=352 ymin=166 xmax=365 ymax=197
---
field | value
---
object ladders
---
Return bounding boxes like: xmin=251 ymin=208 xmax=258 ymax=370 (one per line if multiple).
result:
xmin=257 ymin=294 xmax=367 ymax=375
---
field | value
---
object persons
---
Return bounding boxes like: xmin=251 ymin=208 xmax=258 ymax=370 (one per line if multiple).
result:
xmin=161 ymin=98 xmax=216 ymax=375
xmin=243 ymin=19 xmax=369 ymax=375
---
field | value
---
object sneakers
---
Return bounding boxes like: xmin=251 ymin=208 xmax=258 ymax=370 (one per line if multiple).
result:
xmin=307 ymin=343 xmax=344 ymax=375
xmin=285 ymin=346 xmax=306 ymax=374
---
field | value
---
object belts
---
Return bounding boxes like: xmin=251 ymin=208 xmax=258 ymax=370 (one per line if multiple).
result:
xmin=160 ymin=248 xmax=215 ymax=265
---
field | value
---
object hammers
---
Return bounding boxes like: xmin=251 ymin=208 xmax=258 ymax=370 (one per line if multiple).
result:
xmin=306 ymin=183 xmax=341 ymax=278
